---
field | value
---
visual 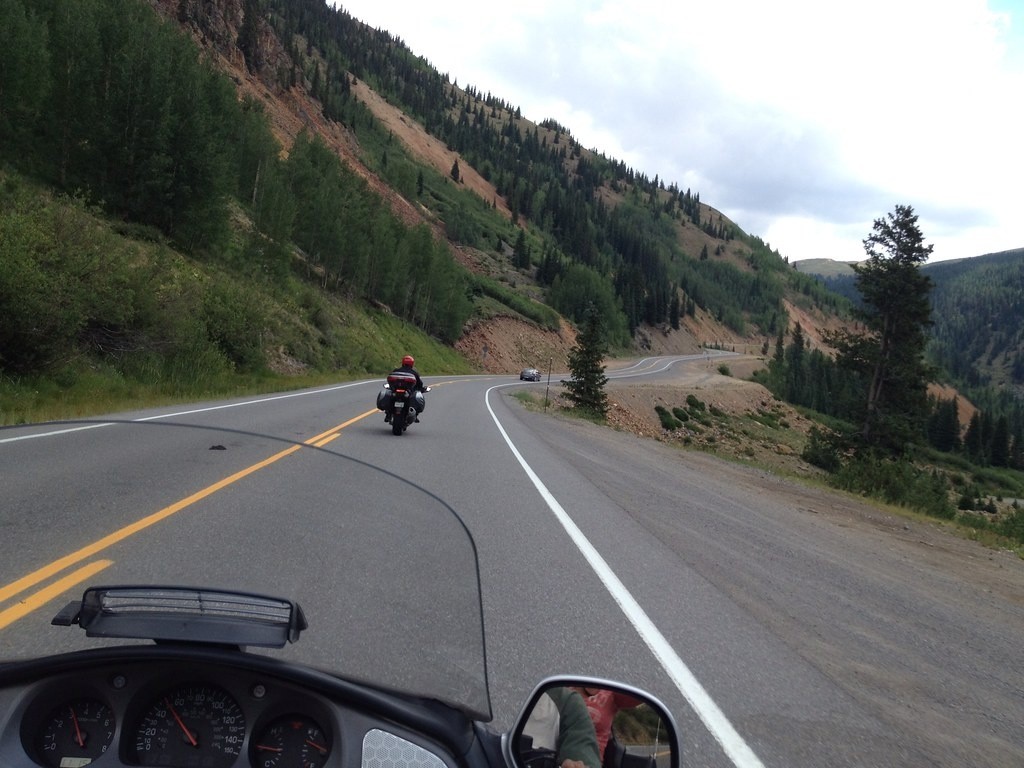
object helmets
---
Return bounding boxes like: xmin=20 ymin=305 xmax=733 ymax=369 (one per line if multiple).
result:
xmin=402 ymin=356 xmax=414 ymax=367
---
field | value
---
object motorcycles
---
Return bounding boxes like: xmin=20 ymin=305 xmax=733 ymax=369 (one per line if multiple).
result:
xmin=376 ymin=371 xmax=432 ymax=436
xmin=0 ymin=419 xmax=680 ymax=768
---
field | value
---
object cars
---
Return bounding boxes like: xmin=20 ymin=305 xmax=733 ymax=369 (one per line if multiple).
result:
xmin=520 ymin=367 xmax=541 ymax=381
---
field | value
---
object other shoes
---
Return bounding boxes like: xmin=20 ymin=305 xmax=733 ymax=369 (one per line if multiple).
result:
xmin=415 ymin=418 xmax=419 ymax=423
xmin=385 ymin=414 xmax=392 ymax=422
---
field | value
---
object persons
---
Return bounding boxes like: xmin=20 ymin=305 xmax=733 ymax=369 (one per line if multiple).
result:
xmin=521 ymin=684 xmax=646 ymax=768
xmin=383 ymin=356 xmax=426 ymax=424
